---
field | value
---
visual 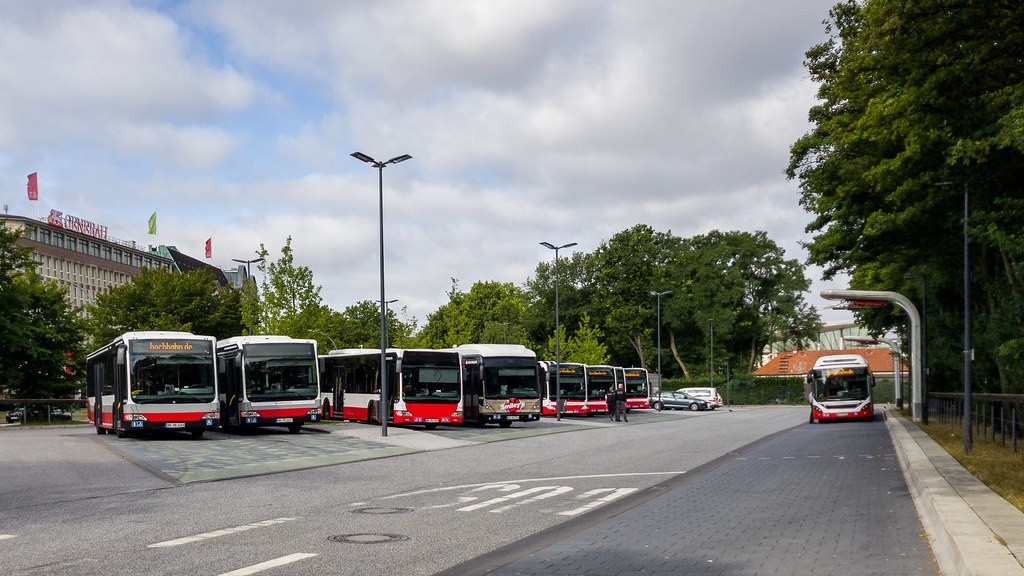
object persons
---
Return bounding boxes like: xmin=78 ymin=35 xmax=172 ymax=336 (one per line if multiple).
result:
xmin=809 ymin=391 xmax=816 ymax=424
xmin=605 ymin=387 xmax=616 ymax=421
xmin=614 ymin=384 xmax=628 ymax=422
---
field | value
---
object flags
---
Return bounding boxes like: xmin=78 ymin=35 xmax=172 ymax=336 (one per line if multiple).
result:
xmin=206 ymin=238 xmax=211 ymax=258
xmin=148 ymin=211 xmax=156 ymax=234
xmin=27 ymin=172 xmax=38 ymax=200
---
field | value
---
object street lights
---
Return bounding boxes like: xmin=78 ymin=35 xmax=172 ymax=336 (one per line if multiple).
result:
xmin=934 ymin=163 xmax=990 ymax=454
xmin=540 ymin=241 xmax=577 ymax=422
xmin=348 ymin=150 xmax=414 ymax=439
xmin=709 ymin=317 xmax=715 ymax=385
xmin=233 ymin=258 xmax=267 ymax=339
xmin=379 ymin=300 xmax=398 ymax=347
xmin=651 ymin=290 xmax=670 ymax=394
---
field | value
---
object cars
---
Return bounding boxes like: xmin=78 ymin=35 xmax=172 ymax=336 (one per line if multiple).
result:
xmin=650 ymin=387 xmax=724 ymax=411
xmin=4 ymin=405 xmax=72 ymax=425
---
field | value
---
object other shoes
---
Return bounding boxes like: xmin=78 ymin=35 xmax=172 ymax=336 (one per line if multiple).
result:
xmin=618 ymin=420 xmax=622 ymax=422
xmin=625 ymin=419 xmax=628 ymax=422
xmin=609 ymin=415 xmax=613 ymax=421
xmin=810 ymin=422 xmax=816 ymax=424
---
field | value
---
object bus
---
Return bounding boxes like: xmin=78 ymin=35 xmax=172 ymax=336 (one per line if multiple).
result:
xmin=539 ymin=361 xmax=651 ymax=418
xmin=453 ymin=344 xmax=542 ymax=428
xmin=217 ymin=336 xmax=322 ymax=435
xmin=805 ymin=355 xmax=877 ymax=421
xmin=318 ymin=348 xmax=462 ymax=428
xmin=85 ymin=330 xmax=221 ymax=439
